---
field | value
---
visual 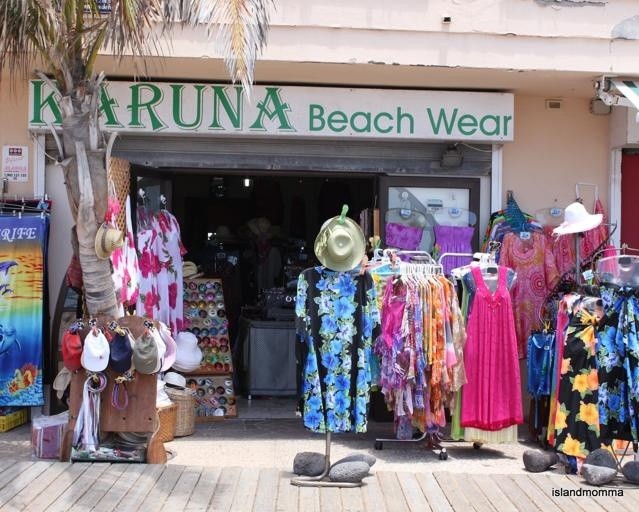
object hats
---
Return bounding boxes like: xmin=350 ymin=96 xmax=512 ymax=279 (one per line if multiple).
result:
xmin=110 ymin=328 xmax=202 ymax=375
xmin=163 ymin=372 xmax=196 ymax=395
xmin=95 ymin=222 xmax=123 ymax=260
xmin=314 ymin=215 xmax=366 ymax=271
xmin=61 ymin=330 xmax=82 ymax=371
xmin=80 ymin=329 xmax=110 ymax=373
xmin=553 ymin=203 xmax=603 ymax=234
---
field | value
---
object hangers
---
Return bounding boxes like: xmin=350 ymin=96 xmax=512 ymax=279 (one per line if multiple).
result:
xmin=364 ymin=197 xmax=531 ymax=288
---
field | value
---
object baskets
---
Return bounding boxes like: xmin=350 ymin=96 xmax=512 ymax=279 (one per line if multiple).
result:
xmin=156 ymin=403 xmax=177 ymax=442
xmin=167 ymin=394 xmax=193 ymax=436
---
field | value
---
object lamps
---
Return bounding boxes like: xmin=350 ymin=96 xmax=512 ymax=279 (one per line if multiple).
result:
xmin=440 ymin=149 xmax=463 ymax=169
